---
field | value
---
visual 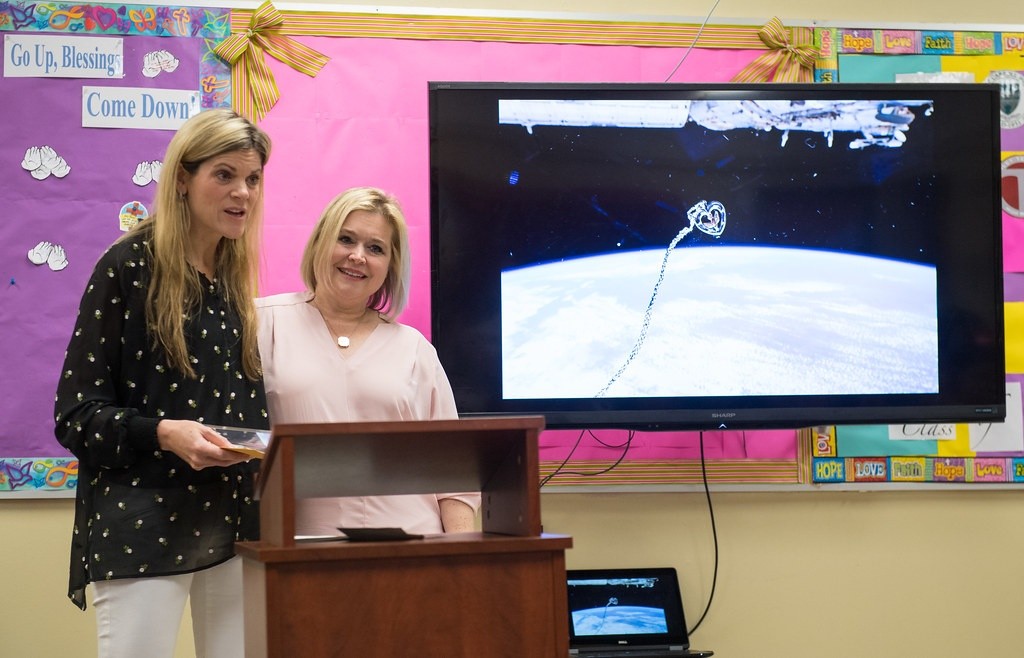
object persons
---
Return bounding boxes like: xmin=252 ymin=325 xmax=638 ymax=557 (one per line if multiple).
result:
xmin=53 ymin=105 xmax=275 ymax=658
xmin=253 ymin=188 xmax=480 ymax=538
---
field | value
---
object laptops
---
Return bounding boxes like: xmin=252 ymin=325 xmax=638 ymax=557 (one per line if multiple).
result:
xmin=565 ymin=568 xmax=715 ymax=658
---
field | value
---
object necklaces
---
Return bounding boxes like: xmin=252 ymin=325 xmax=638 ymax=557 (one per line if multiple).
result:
xmin=311 ymin=297 xmax=365 ymax=348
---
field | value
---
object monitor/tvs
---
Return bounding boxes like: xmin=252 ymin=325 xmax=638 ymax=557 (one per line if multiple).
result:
xmin=428 ymin=83 xmax=1007 ymax=432
